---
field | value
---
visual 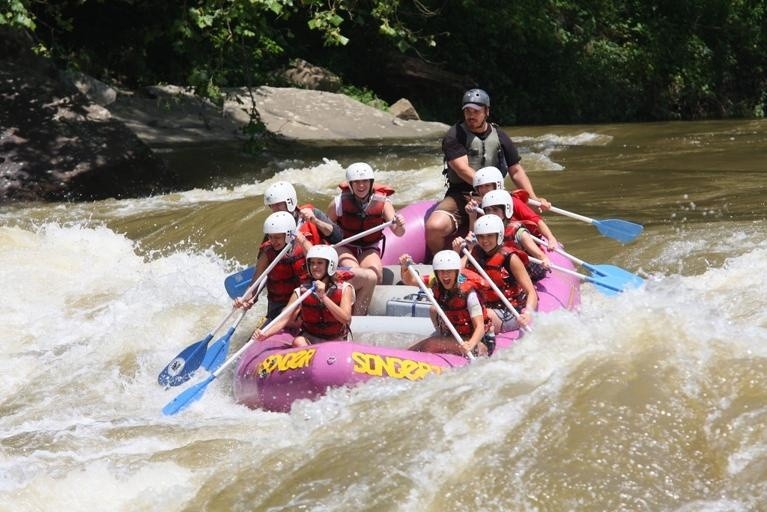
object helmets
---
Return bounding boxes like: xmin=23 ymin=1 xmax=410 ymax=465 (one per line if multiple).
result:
xmin=481 ymin=189 xmax=514 ymax=219
xmin=472 ymin=215 xmax=505 ymax=247
xmin=344 ymin=162 xmax=376 ymax=197
xmin=263 ymin=181 xmax=298 ymax=213
xmin=432 ymin=249 xmax=462 ymax=289
xmin=305 ymin=244 xmax=339 ymax=279
xmin=462 ymin=88 xmax=490 ymax=111
xmin=472 ymin=165 xmax=505 ymax=192
xmin=262 ymin=210 xmax=296 ymax=245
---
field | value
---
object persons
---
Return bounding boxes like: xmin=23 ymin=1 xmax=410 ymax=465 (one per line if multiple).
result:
xmin=451 ymin=213 xmax=539 ymax=334
xmin=250 ymin=244 xmax=356 ymax=349
xmin=325 ymin=162 xmax=407 ymax=286
xmin=464 ymin=189 xmax=552 ymax=280
xmin=261 ymin=180 xmax=345 ymax=247
xmin=424 ymin=88 xmax=552 ymax=257
xmin=397 ymin=249 xmax=497 ymax=357
xmin=464 ymin=165 xmax=560 ymax=254
xmin=231 ymin=210 xmax=378 ymax=321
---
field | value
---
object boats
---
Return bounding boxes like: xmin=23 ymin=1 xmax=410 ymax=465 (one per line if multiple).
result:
xmin=234 ymin=199 xmax=581 ymax=410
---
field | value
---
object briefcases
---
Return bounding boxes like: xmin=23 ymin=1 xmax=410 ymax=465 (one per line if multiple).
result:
xmin=385 ymin=292 xmax=433 ymax=317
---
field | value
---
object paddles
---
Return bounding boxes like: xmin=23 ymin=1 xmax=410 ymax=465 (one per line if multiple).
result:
xmin=467 ymin=232 xmax=625 ymax=296
xmin=201 ymin=212 xmax=312 ymax=372
xmin=225 ymin=215 xmax=404 ymax=300
xmin=158 ymin=232 xmax=297 ymax=386
xmin=162 ymin=281 xmax=317 ymax=417
xmin=528 ymin=197 xmax=642 ymax=243
xmin=468 ymin=203 xmax=643 ymax=288
